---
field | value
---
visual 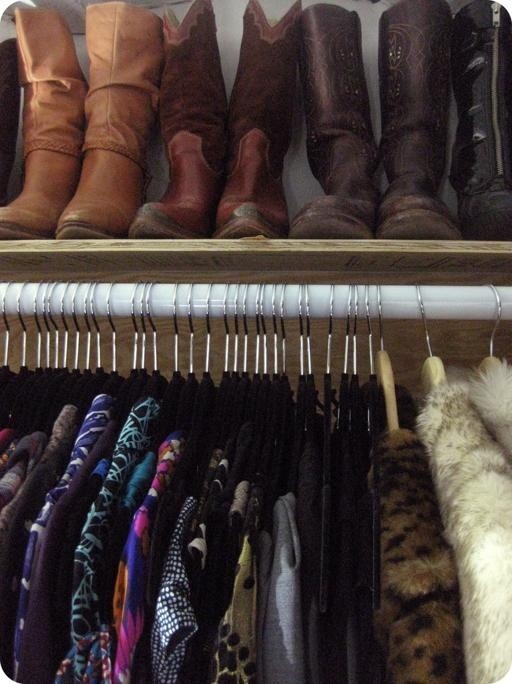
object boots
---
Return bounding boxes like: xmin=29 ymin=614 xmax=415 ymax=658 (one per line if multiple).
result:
xmin=127 ymin=0 xmax=229 ymax=240
xmin=451 ymin=1 xmax=512 ymax=241
xmin=287 ymin=3 xmax=384 ymax=240
xmin=213 ymin=1 xmax=306 ymax=239
xmin=0 ymin=6 xmax=90 ymax=239
xmin=376 ymin=1 xmax=462 ymax=241
xmin=55 ymin=2 xmax=164 ymax=240
